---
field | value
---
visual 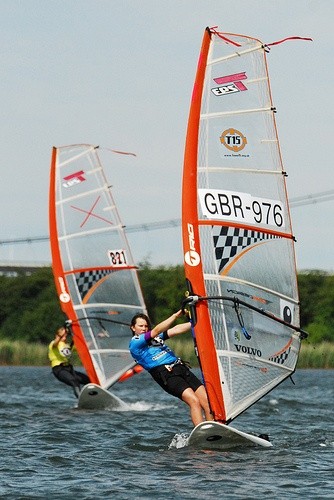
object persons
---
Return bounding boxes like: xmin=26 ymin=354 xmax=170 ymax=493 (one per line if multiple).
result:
xmin=47 ymin=325 xmax=91 ymax=398
xmin=128 ymin=308 xmax=214 ymax=427
